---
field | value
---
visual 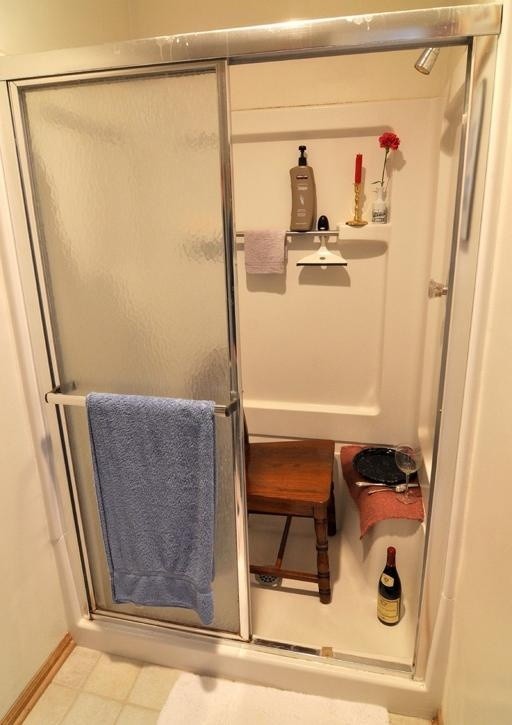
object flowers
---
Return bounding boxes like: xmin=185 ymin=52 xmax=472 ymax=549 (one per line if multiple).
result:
xmin=370 ymin=132 xmax=400 ymax=187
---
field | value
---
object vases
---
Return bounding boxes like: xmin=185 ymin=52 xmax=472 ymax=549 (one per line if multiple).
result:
xmin=372 ymin=187 xmax=388 ymax=224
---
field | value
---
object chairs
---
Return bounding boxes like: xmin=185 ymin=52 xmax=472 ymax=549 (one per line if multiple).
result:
xmin=244 ymin=410 xmax=335 ymax=604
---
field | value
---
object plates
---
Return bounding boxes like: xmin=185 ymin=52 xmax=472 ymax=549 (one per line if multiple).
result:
xmin=352 ymin=446 xmax=416 ymax=485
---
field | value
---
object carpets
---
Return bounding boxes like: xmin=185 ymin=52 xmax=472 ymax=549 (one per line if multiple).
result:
xmin=156 ymin=672 xmax=389 ymax=725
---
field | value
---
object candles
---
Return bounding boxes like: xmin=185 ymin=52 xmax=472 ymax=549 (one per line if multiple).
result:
xmin=355 ymin=153 xmax=362 ymax=184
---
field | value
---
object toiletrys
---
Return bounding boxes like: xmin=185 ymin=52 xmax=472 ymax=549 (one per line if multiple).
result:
xmin=289 ymin=145 xmax=317 ymax=232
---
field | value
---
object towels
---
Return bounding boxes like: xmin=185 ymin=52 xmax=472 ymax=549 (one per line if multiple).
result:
xmin=86 ymin=391 xmax=216 ymax=625
xmin=243 ymin=227 xmax=289 ymax=274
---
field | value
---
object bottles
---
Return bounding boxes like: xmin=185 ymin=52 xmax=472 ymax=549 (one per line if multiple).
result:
xmin=377 ymin=547 xmax=404 ymax=626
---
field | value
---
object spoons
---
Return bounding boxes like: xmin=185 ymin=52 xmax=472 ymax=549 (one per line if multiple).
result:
xmin=365 ymin=482 xmax=407 ymax=497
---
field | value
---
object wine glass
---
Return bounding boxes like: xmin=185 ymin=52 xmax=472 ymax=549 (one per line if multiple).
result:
xmin=395 ymin=439 xmax=421 ymax=504
